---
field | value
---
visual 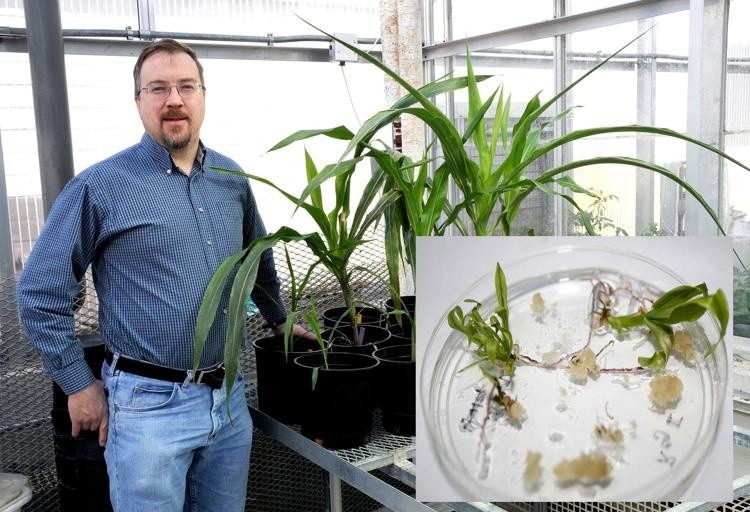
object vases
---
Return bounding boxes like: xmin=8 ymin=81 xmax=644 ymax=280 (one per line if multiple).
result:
xmin=324 ymin=306 xmax=384 ymax=324
xmin=385 ymin=293 xmax=417 ymax=314
xmin=373 ymin=345 xmax=418 ymax=440
xmin=391 ymin=323 xmax=416 ymax=344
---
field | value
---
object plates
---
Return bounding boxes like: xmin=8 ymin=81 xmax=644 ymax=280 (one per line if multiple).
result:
xmin=421 ymin=246 xmax=728 ymax=503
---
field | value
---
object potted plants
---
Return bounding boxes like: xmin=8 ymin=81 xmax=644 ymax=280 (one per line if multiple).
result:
xmin=250 ymin=238 xmax=374 ymax=420
xmin=281 ymin=290 xmax=380 ymax=450
xmin=191 ymin=146 xmax=389 ymax=414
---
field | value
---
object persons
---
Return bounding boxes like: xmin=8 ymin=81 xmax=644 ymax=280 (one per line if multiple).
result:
xmin=15 ymin=40 xmax=316 ymax=512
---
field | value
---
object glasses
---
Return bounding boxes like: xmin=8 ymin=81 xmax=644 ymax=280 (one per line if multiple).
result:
xmin=136 ymin=79 xmax=206 ymax=99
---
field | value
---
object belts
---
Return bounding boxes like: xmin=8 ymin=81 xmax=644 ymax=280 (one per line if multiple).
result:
xmin=104 ymin=347 xmax=227 ymax=389
